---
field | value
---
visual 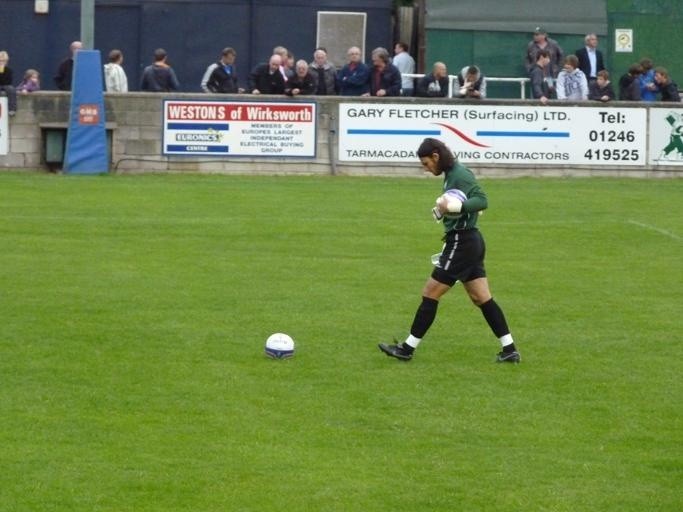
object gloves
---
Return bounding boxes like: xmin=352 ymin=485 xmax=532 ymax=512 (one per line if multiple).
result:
xmin=437 ymin=192 xmax=462 ymax=213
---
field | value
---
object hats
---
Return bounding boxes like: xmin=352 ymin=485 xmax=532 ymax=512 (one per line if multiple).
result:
xmin=531 ymin=25 xmax=547 ymax=35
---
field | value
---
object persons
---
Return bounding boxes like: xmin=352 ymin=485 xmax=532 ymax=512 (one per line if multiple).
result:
xmin=524 ymin=24 xmax=681 ymax=105
xmin=16 ymin=68 xmax=41 ymax=94
xmin=246 ymin=41 xmax=487 ymax=98
xmin=103 ymin=49 xmax=129 ymax=92
xmin=378 ymin=137 xmax=523 ymax=365
xmin=0 ymin=50 xmax=13 ymax=88
xmin=199 ymin=46 xmax=245 ymax=93
xmin=52 ymin=40 xmax=82 ymax=91
xmin=139 ymin=47 xmax=181 ymax=91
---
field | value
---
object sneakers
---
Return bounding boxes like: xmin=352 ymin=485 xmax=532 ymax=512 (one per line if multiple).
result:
xmin=496 ymin=352 xmax=521 ymax=365
xmin=378 ymin=343 xmax=414 ymax=361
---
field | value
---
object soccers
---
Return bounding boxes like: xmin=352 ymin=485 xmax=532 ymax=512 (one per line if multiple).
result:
xmin=440 ymin=188 xmax=466 ymax=219
xmin=265 ymin=332 xmax=294 ymax=359
